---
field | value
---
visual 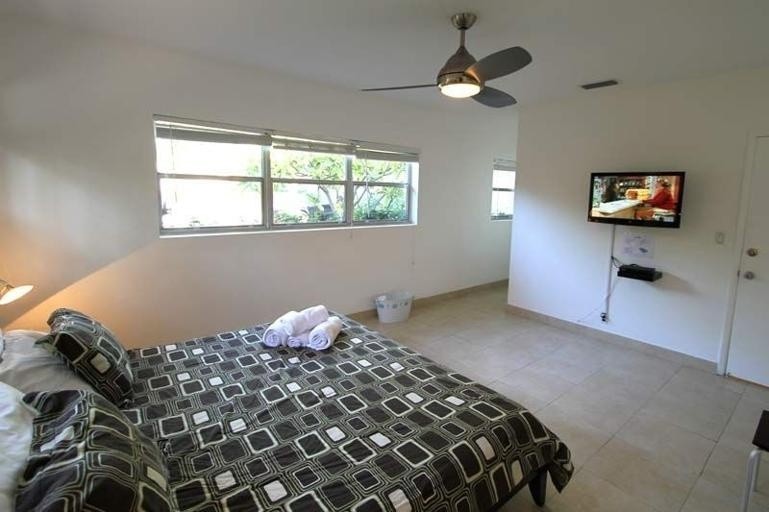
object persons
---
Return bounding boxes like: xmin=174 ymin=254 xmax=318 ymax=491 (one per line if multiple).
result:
xmin=636 ymin=178 xmax=675 ymax=221
xmin=605 ymin=182 xmax=625 ymax=202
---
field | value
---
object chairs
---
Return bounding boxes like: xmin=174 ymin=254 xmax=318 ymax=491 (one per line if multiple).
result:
xmin=741 ymin=407 xmax=769 ymax=512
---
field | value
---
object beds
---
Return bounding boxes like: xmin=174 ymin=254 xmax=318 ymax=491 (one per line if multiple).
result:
xmin=121 ymin=306 xmax=576 ymax=511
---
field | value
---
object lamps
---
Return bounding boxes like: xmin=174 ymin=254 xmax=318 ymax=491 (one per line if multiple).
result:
xmin=437 ymin=72 xmax=484 ymax=102
xmin=0 ymin=274 xmax=35 ymax=305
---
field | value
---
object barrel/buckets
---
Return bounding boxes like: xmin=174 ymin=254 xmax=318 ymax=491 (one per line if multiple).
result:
xmin=372 ymin=289 xmax=414 ymax=323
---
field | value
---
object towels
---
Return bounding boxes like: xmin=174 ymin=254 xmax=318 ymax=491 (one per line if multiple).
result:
xmin=264 ymin=304 xmax=343 ymax=352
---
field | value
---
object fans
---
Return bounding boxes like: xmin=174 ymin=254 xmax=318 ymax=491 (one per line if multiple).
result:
xmin=358 ymin=9 xmax=535 ymax=110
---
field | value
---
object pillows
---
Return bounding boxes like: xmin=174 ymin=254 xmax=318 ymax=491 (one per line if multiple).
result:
xmin=34 ymin=307 xmax=136 ymax=402
xmin=13 ymin=389 xmax=174 ymax=511
xmin=0 ymin=326 xmax=98 ymax=394
xmin=0 ymin=379 xmax=43 ymax=512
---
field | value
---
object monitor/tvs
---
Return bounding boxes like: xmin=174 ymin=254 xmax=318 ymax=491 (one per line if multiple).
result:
xmin=587 ymin=171 xmax=685 ymax=228
